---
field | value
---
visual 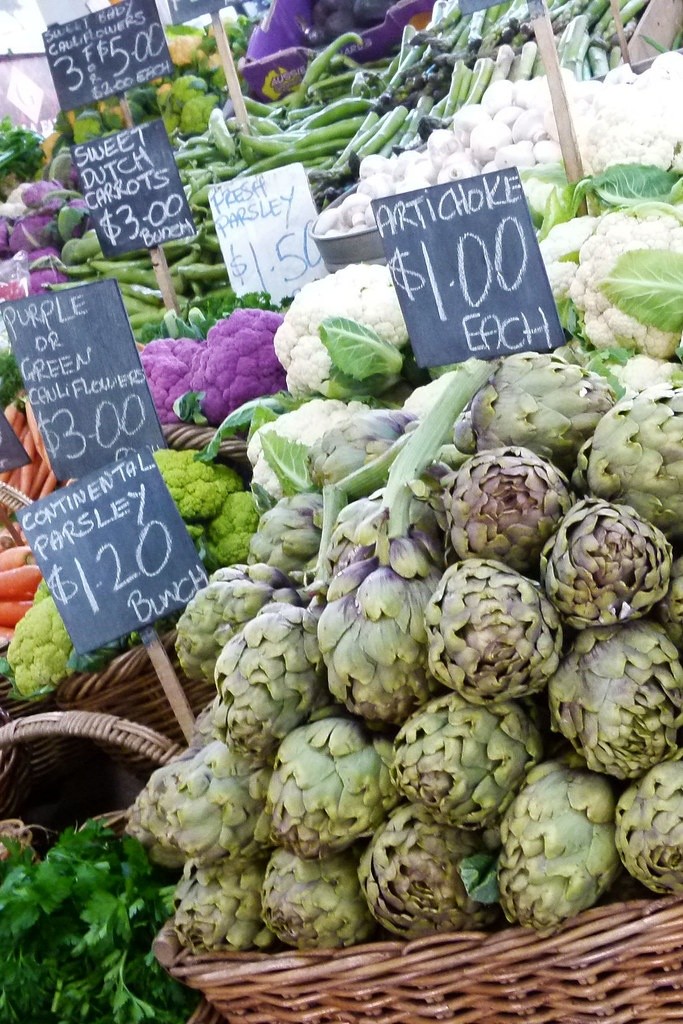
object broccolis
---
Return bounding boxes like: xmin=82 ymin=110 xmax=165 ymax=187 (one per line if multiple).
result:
xmin=0 ymin=14 xmax=251 ymax=341
xmin=574 ymin=74 xmax=683 ymax=178
xmin=6 ymin=579 xmax=187 ymax=704
xmin=138 ymin=161 xmax=683 ymax=572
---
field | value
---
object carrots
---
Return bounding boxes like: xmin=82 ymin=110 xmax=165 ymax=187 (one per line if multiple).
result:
xmin=0 ymin=399 xmax=68 ymax=641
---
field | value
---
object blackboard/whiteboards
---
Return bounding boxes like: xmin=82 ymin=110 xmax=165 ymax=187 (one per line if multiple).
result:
xmin=14 ymin=446 xmax=209 ymax=656
xmin=71 ymin=118 xmax=196 ymax=260
xmin=1 ymin=279 xmax=168 ymax=482
xmin=167 ymin=0 xmax=248 ymax=27
xmin=371 ymin=167 xmax=567 ymax=368
xmin=207 ymin=162 xmax=330 ymax=307
xmin=41 ymin=0 xmax=175 ymax=113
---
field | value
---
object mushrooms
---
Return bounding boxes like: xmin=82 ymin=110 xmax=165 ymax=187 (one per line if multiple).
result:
xmin=317 ymin=49 xmax=683 ymax=238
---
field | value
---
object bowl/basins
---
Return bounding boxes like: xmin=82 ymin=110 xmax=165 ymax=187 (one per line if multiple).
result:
xmin=306 ymin=143 xmax=428 ymax=274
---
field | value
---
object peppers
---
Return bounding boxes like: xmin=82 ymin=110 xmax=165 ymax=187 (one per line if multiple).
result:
xmin=49 ymin=31 xmax=386 ymax=334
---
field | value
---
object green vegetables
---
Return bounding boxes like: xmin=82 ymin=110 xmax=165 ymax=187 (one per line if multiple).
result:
xmin=0 ymin=292 xmax=300 ymax=412
xmin=0 ymin=816 xmax=209 ymax=1024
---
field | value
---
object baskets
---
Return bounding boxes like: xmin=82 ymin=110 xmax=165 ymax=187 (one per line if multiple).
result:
xmin=56 ymin=631 xmax=217 ymax=740
xmin=0 ymin=711 xmax=221 ymax=1024
xmin=0 ymin=481 xmax=56 ymax=720
xmin=159 ymin=422 xmax=248 ymax=463
xmin=171 ymin=890 xmax=683 ymax=1024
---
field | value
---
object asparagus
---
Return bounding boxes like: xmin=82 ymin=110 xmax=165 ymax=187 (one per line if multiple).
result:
xmin=310 ymin=0 xmax=650 ymax=201
